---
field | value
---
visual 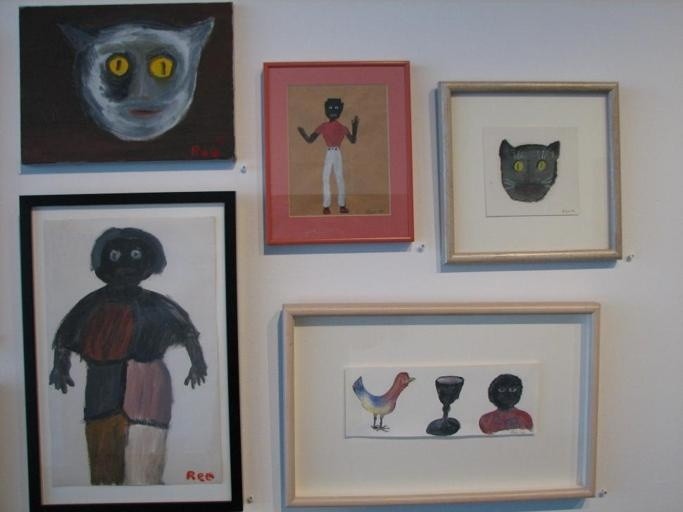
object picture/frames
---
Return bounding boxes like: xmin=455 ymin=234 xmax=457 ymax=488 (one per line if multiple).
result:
xmin=15 ymin=4 xmax=239 ymax=167
xmin=18 ymin=192 xmax=247 ymax=511
xmin=257 ymin=63 xmax=419 ymax=246
xmin=277 ymin=297 xmax=601 ymax=511
xmin=432 ymin=78 xmax=619 ymax=269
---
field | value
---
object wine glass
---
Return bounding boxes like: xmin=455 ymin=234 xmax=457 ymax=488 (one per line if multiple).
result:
xmin=425 ymin=375 xmax=463 ymax=435
